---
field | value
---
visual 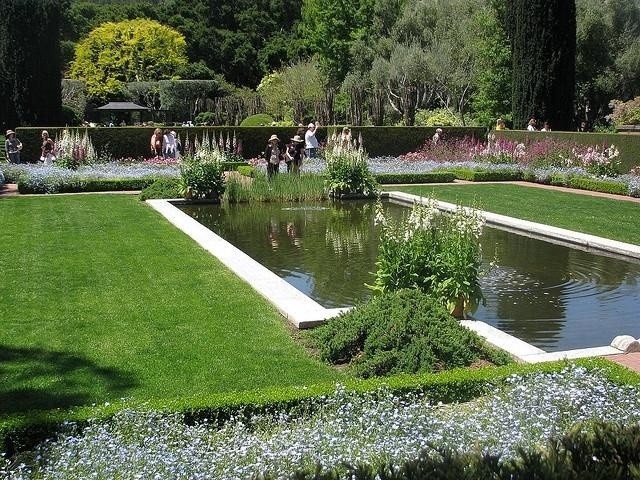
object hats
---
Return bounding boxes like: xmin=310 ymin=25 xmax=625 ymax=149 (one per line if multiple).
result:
xmin=268 ymin=135 xmax=279 ymax=142
xmin=290 ymin=134 xmax=303 ymax=142
xmin=308 ymin=123 xmax=315 ymax=128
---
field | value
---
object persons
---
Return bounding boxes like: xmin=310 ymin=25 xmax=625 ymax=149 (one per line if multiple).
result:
xmin=150 ymin=128 xmax=181 ymax=159
xmin=285 ymin=135 xmax=307 ymax=172
xmin=265 ymin=134 xmax=285 ymax=173
xmin=182 ymin=120 xmax=194 ymax=127
xmin=342 ymin=126 xmax=349 ymax=137
xmin=40 ymin=130 xmax=56 ymax=164
xmin=496 ymin=114 xmax=506 ymax=130
xmin=305 ymin=121 xmax=319 ymax=158
xmin=109 ymin=117 xmax=126 ymax=127
xmin=433 ymin=128 xmax=443 ymax=143
xmin=4 ymin=129 xmax=23 ymax=163
xmin=296 ymin=123 xmax=307 ymax=158
xmin=526 ymin=119 xmax=538 ymax=131
xmin=541 ymin=121 xmax=553 ymax=132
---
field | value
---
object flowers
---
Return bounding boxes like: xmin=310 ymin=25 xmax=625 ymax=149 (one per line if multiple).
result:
xmin=175 ymin=129 xmax=244 ymax=200
xmin=324 ymin=128 xmax=382 ymax=197
xmin=364 ymin=188 xmax=487 ymax=320
